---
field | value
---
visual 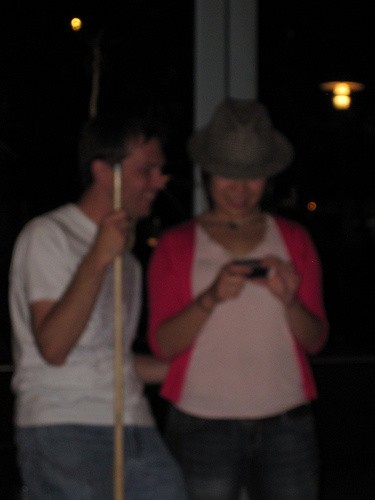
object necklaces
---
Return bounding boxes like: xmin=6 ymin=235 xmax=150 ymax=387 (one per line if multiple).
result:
xmin=213 ymin=212 xmax=260 ymax=229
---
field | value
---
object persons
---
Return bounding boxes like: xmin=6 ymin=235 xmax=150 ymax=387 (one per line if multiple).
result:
xmin=146 ymin=98 xmax=331 ymax=500
xmin=6 ymin=116 xmax=188 ymax=500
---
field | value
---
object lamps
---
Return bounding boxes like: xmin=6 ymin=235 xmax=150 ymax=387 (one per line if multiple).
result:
xmin=318 ymin=79 xmax=362 ymax=110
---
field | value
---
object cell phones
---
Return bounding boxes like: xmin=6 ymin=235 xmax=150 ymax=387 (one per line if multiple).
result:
xmin=231 ymin=257 xmax=269 ymax=277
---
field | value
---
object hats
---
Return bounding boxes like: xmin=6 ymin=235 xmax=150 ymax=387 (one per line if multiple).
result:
xmin=191 ymin=96 xmax=293 ymax=177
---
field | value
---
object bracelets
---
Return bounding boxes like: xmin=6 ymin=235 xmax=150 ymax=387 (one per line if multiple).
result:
xmin=197 ymin=296 xmax=211 ymax=310
